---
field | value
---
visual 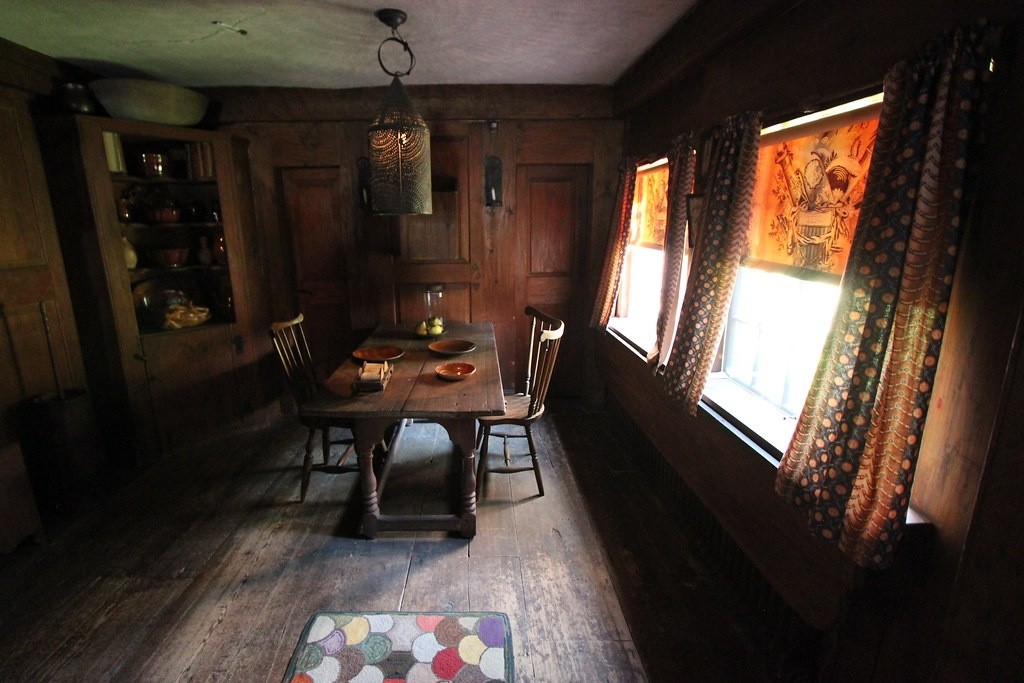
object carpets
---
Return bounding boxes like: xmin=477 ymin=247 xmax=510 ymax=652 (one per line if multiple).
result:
xmin=282 ymin=610 xmax=515 ymax=683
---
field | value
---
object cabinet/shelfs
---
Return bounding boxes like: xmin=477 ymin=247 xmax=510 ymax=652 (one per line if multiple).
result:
xmin=32 ymin=113 xmax=292 ymax=465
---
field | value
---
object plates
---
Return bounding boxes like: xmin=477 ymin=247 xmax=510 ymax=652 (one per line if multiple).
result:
xmin=352 ymin=345 xmax=405 ymax=362
xmin=428 ymin=339 xmax=477 ymax=354
xmin=416 ymin=326 xmax=449 ymax=336
xmin=434 ymin=362 xmax=476 ymax=380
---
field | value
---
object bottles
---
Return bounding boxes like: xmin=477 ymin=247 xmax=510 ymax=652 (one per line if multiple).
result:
xmin=198 ymin=236 xmax=214 ymax=266
xmin=149 ymin=228 xmax=190 ymax=269
xmin=121 ymin=235 xmax=139 ymax=270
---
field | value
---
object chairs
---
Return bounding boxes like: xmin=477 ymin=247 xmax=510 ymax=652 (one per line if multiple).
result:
xmin=477 ymin=306 xmax=565 ymax=501
xmin=268 ymin=312 xmax=390 ymax=502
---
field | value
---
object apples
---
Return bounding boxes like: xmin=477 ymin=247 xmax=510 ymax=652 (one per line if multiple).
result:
xmin=415 ymin=316 xmax=443 ymax=334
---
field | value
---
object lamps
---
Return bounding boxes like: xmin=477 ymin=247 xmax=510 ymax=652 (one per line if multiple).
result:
xmin=366 ymin=7 xmax=433 ymax=216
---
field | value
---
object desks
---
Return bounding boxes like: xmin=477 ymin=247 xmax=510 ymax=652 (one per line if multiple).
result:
xmin=297 ymin=322 xmax=506 ymax=540
